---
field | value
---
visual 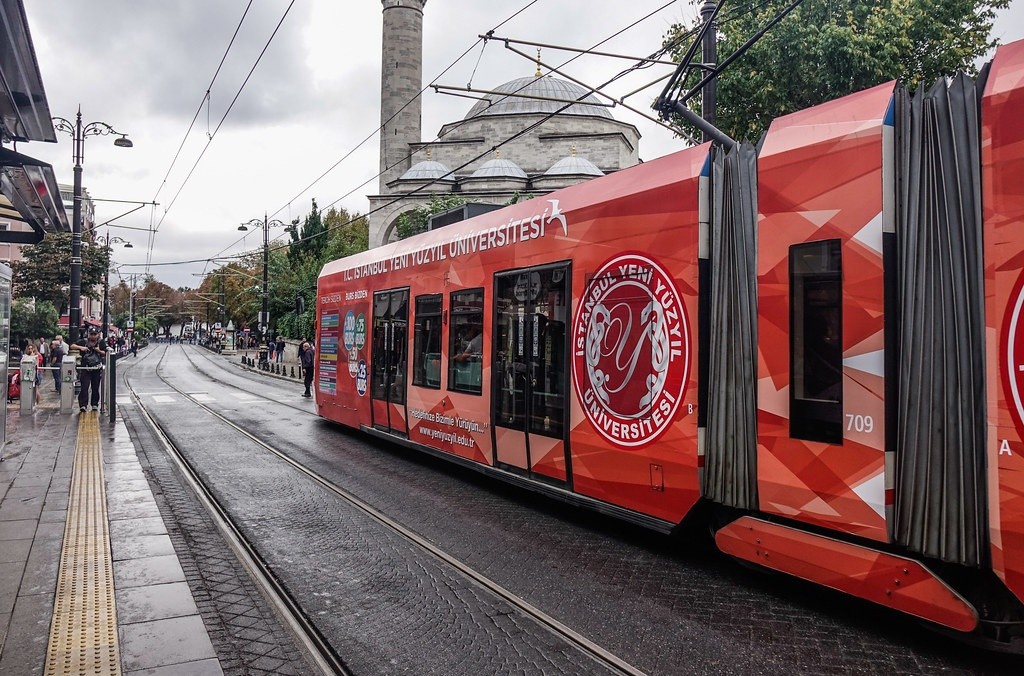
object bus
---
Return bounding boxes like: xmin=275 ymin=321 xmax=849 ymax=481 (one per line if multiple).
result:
xmin=311 ymin=0 xmax=1024 ymax=676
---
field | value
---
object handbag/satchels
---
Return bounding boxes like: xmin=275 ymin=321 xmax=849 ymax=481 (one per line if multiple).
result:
xmin=82 ymin=351 xmax=102 ymax=366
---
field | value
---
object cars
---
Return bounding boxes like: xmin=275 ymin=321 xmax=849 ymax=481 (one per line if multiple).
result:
xmin=183 ymin=324 xmax=206 ymax=339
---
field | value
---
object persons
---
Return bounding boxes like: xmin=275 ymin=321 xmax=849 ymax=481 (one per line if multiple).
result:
xmin=457 ymin=326 xmax=485 ymax=365
xmin=173 ymin=335 xmax=178 ymax=340
xmin=248 ymin=336 xmax=259 ymax=349
xmin=108 ymin=335 xmax=126 ymax=350
xmin=296 ymin=337 xmax=314 ymax=397
xmin=276 ymin=337 xmax=285 ymax=363
xmin=70 ymin=327 xmax=108 ymax=412
xmin=131 ymin=339 xmax=138 ymax=357
xmin=269 ymin=340 xmax=274 ymax=359
xmin=26 ymin=335 xmax=69 ymax=394
xmin=215 ymin=333 xmax=227 ymax=348
xmin=187 ymin=335 xmax=192 ymax=344
xmin=240 ymin=338 xmax=244 ymax=349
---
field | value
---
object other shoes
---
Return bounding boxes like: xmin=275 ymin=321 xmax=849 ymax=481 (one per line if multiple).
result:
xmin=92 ymin=406 xmax=98 ymax=410
xmin=51 ymin=389 xmax=58 ymax=392
xmin=301 ymin=393 xmax=311 ymax=397
xmin=80 ymin=406 xmax=88 ymax=413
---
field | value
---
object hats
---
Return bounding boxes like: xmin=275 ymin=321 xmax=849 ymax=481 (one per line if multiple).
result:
xmin=88 ymin=325 xmax=101 ymax=334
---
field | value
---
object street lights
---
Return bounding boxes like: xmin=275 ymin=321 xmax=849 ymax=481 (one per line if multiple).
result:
xmin=91 ymin=228 xmax=134 ymax=339
xmin=237 ymin=211 xmax=294 ymax=372
xmin=51 ymin=102 xmax=134 ymax=394
xmin=208 ymin=266 xmax=232 ymax=327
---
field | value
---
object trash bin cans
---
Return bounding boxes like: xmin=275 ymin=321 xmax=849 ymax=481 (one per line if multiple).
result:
xmin=260 ymin=351 xmax=268 ymax=364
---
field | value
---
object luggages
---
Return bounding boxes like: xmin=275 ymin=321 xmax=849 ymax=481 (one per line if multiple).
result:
xmin=8 ymin=371 xmax=21 ymax=403
